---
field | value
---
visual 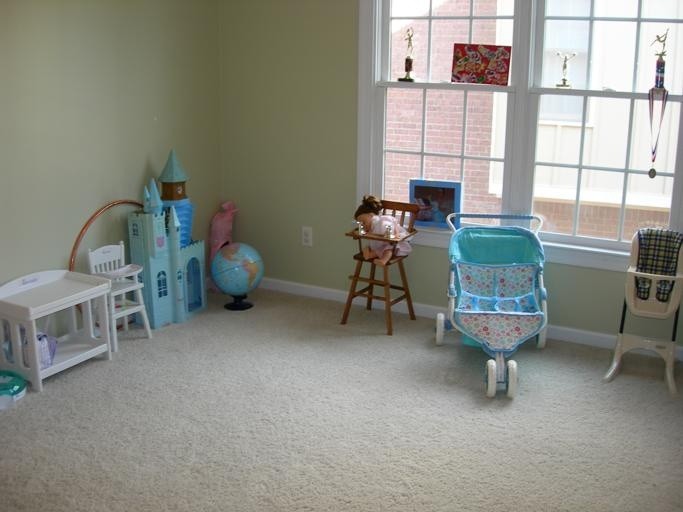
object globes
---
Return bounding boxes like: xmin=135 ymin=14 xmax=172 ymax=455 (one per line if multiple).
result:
xmin=210 ymin=240 xmax=264 ymax=310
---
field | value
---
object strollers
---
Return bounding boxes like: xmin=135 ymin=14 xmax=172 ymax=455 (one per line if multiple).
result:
xmin=433 ymin=212 xmax=554 ymax=401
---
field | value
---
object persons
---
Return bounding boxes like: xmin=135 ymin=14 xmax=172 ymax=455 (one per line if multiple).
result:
xmin=353 ymin=195 xmax=412 ymax=267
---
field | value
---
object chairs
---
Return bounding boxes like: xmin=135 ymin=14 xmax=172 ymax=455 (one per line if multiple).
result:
xmin=86 ymin=243 xmax=158 ymax=353
xmin=341 ymin=199 xmax=419 ymax=336
xmin=598 ymin=227 xmax=682 ymax=394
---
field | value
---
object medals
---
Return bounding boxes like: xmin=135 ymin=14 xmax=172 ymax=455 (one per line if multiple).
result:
xmin=647 ymin=168 xmax=656 ymax=178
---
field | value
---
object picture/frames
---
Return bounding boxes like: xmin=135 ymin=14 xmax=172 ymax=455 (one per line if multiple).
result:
xmin=409 ymin=178 xmax=465 ymax=227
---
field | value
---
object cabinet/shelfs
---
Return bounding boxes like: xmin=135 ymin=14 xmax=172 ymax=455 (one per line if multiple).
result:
xmin=0 ymin=268 xmax=118 ymax=391
